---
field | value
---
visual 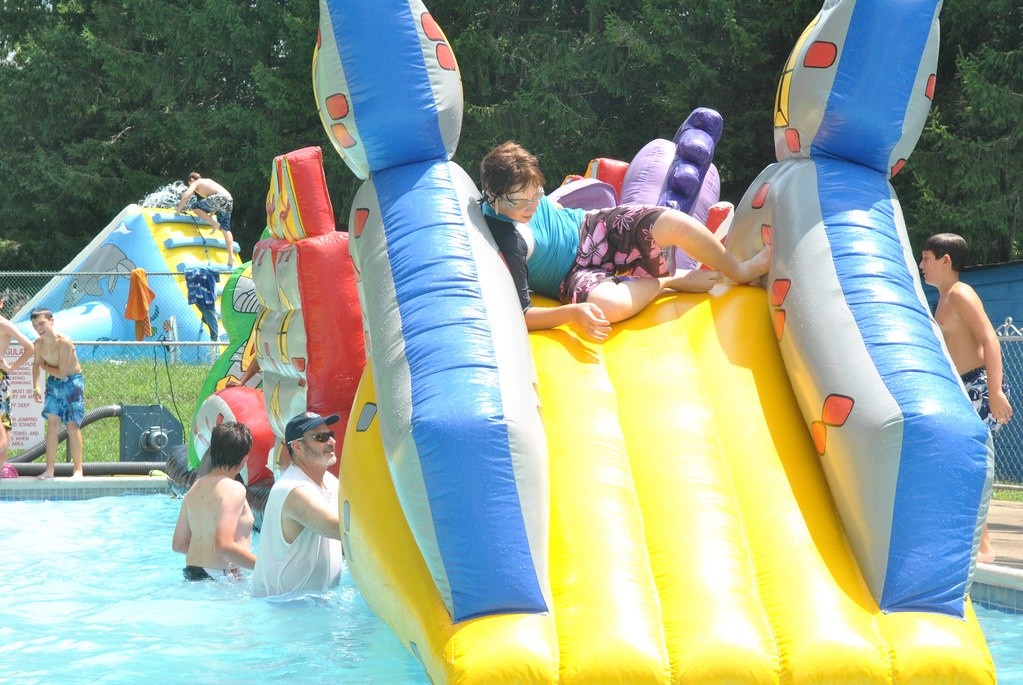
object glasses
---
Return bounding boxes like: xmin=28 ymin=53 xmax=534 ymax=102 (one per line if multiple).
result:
xmin=287 ymin=430 xmax=334 ymax=445
xmin=495 ymin=185 xmax=545 ymax=215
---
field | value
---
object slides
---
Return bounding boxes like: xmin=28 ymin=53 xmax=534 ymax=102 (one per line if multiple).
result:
xmin=311 ymin=159 xmax=1003 ymax=684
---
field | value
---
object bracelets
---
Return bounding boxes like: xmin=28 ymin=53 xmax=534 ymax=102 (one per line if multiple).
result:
xmin=33 ymin=387 xmax=39 ymax=392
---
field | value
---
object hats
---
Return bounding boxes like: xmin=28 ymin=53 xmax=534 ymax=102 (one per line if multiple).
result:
xmin=285 ymin=411 xmax=340 ymax=442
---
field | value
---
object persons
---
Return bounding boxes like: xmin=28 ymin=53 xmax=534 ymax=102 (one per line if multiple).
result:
xmin=480 ymin=142 xmax=772 ymax=340
xmin=249 ymin=412 xmax=342 ymax=597
xmin=176 ymin=172 xmax=234 ymax=265
xmin=0 ymin=293 xmax=34 ymax=466
xmin=172 ymin=420 xmax=256 ymax=578
xmin=31 ymin=308 xmax=85 ymax=480
xmin=919 ymin=233 xmax=1013 ymax=564
